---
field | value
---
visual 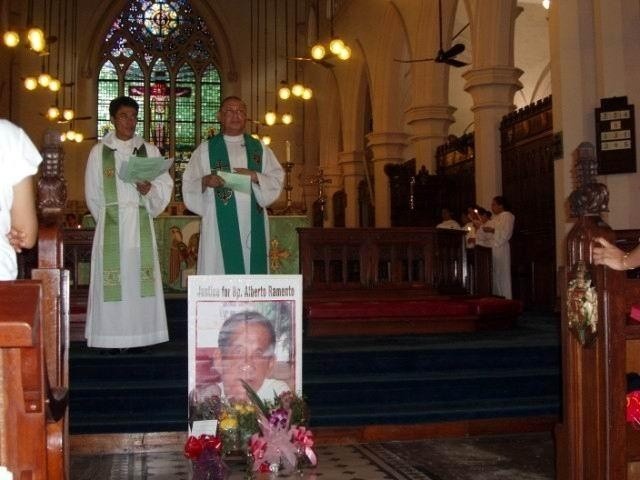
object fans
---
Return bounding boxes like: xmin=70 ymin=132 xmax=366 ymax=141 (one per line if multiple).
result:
xmin=391 ymin=0 xmax=476 ymax=69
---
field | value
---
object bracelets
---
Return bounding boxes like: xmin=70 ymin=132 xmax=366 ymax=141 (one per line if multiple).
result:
xmin=622 ymin=252 xmax=637 ymax=271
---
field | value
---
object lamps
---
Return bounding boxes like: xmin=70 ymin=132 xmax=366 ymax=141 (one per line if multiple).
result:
xmin=0 ymin=0 xmax=84 ymax=143
xmin=309 ymin=1 xmax=352 ymax=62
xmin=247 ymin=2 xmax=292 ymax=145
xmin=277 ymin=0 xmax=314 ymax=102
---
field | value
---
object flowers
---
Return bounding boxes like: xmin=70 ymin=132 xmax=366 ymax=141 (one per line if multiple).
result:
xmin=177 ymin=378 xmax=318 ymax=478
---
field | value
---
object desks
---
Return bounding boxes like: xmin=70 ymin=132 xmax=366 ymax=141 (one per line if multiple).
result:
xmin=294 ymin=225 xmax=468 ymax=297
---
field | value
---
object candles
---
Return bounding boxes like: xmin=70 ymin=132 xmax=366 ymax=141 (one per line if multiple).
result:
xmin=286 ymin=140 xmax=291 ymax=162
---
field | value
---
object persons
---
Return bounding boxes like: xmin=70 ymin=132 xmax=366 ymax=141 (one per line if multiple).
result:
xmin=198 ymin=310 xmax=292 ymax=408
xmin=84 ymin=95 xmax=173 ymax=353
xmin=181 ymin=94 xmax=286 ymax=275
xmin=591 ymin=234 xmax=640 ymax=271
xmin=63 ymin=214 xmax=79 ymax=229
xmin=434 ymin=194 xmax=515 ymax=301
xmin=1 ymin=118 xmax=44 ymax=282
